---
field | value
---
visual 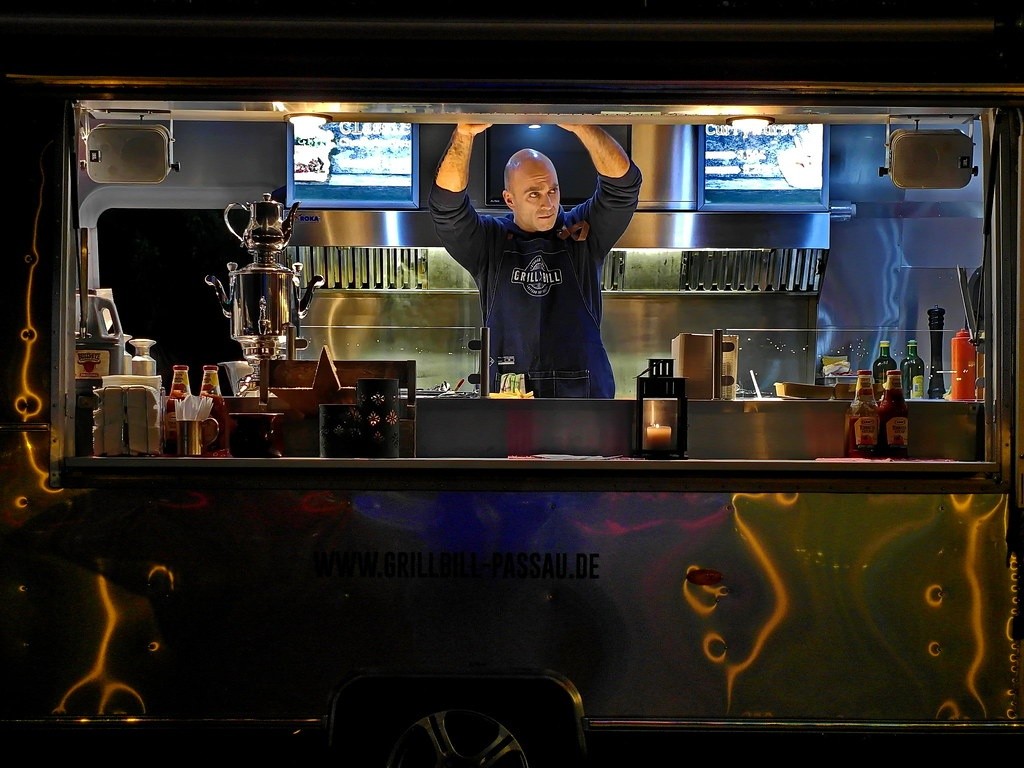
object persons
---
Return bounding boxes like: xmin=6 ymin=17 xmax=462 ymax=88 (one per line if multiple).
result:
xmin=427 ymin=124 xmax=643 ymax=399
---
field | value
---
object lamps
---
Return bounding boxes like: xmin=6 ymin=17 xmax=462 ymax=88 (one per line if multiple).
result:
xmin=879 ymin=113 xmax=978 ymax=191
xmin=726 ymin=116 xmax=774 ymax=132
xmin=646 ymin=424 xmax=672 ymax=451
xmin=283 ymin=113 xmax=334 ymax=127
xmin=82 ymin=111 xmax=179 ymax=184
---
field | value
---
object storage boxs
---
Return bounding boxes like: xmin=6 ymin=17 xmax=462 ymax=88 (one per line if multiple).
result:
xmin=670 ymin=332 xmax=738 ymax=401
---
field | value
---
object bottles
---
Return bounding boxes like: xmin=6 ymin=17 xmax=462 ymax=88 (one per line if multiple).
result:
xmin=872 ymin=341 xmax=897 ymax=383
xmin=951 ymin=329 xmax=976 ymax=399
xmin=128 ymin=338 xmax=157 ymax=376
xmin=900 ymin=340 xmax=924 ymax=399
xmin=74 ymin=290 xmax=124 ymax=409
xmin=879 ymin=370 xmax=909 ymax=458
xmin=228 ymin=412 xmax=284 ymax=458
xmin=110 ymin=332 xmax=132 ymax=375
xmin=163 ymin=365 xmax=191 ymax=454
xmin=199 ymin=365 xmax=226 ymax=456
xmin=844 ymin=370 xmax=879 ymax=457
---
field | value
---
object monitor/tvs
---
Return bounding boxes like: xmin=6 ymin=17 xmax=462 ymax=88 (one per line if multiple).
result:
xmin=484 ymin=122 xmax=632 ymax=210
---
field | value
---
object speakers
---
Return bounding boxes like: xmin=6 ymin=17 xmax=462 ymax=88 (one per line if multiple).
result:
xmin=86 ymin=123 xmax=171 ymax=184
xmin=890 ymin=127 xmax=973 ymax=190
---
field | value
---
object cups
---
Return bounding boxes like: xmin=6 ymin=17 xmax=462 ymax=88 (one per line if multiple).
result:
xmin=318 ymin=404 xmax=356 ymax=459
xmin=176 ymin=418 xmax=219 ymax=456
xmin=356 ymin=378 xmax=400 ymax=458
